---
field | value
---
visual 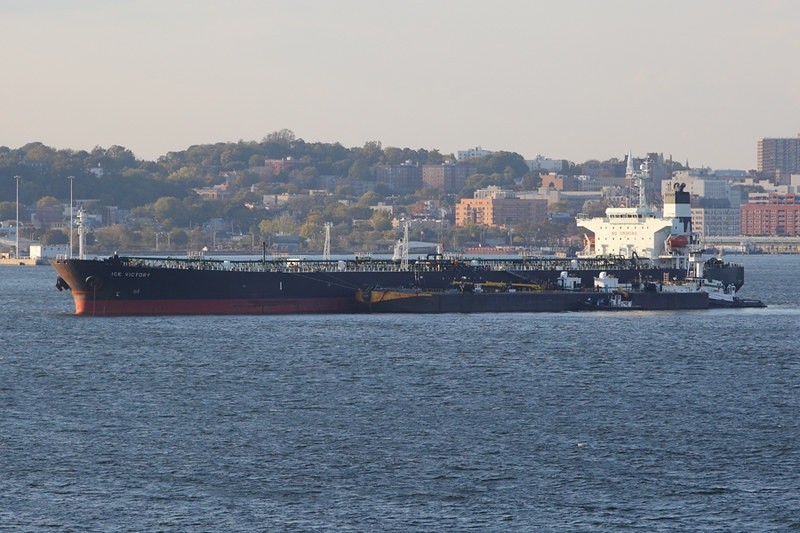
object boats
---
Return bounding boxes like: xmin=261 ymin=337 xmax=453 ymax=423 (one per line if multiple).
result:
xmin=666 ymin=234 xmax=689 ymax=248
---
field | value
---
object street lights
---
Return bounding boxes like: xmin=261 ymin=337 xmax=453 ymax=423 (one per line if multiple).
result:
xmin=67 ymin=176 xmax=77 ymax=260
xmin=14 ymin=176 xmax=23 ymax=259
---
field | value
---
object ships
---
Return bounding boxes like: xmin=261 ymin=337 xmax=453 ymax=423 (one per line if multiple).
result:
xmin=46 ymin=150 xmax=768 ymax=317
